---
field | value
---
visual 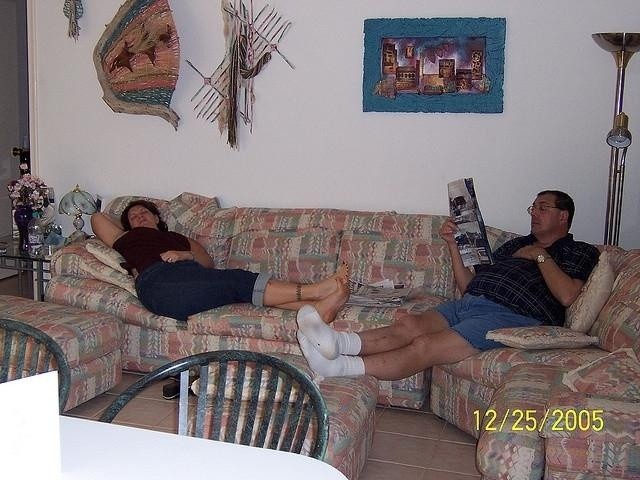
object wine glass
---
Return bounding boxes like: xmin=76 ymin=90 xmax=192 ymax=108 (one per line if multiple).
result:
xmin=167 ymin=257 xmax=173 ymax=262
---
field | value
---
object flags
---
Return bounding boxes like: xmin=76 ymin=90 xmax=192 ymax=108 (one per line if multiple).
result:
xmin=12 ymin=205 xmax=33 ymax=253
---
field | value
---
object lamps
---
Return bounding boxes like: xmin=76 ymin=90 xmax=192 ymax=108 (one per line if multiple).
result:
xmin=0 ymin=315 xmax=73 ymax=417
xmin=93 ymin=350 xmax=332 ymax=465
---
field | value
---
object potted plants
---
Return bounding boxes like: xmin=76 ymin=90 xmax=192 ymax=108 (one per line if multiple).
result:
xmin=359 ymin=11 xmax=507 ymax=117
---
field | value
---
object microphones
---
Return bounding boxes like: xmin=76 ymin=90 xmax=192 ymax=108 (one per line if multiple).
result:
xmin=176 ymin=349 xmax=383 ymax=480
xmin=471 ymin=356 xmax=640 ymax=479
xmin=0 ymin=292 xmax=126 ymax=416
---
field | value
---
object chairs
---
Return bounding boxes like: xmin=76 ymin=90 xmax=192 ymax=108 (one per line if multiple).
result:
xmin=161 ymin=372 xmax=201 ymax=401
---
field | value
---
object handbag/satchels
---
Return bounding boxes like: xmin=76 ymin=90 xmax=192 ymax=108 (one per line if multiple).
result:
xmin=189 ymin=250 xmax=195 ymax=263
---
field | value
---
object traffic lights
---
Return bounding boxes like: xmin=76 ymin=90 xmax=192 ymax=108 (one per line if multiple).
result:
xmin=187 ymin=339 xmax=325 ymax=408
xmin=84 ymin=236 xmax=133 ymax=280
xmin=76 ymin=255 xmax=142 ymax=301
xmin=559 ymin=248 xmax=615 ymax=339
xmin=556 ymin=343 xmax=639 ymax=402
xmin=481 ymin=318 xmax=603 ymax=351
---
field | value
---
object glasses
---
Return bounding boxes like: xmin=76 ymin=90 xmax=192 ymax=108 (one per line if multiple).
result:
xmin=56 ymin=182 xmax=99 ymax=242
xmin=587 ymin=30 xmax=640 ymax=250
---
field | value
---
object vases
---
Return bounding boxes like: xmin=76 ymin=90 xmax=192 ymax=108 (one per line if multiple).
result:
xmin=6 ymin=171 xmax=59 ymax=211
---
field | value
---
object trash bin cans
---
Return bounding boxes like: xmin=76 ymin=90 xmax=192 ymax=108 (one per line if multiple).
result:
xmin=535 ymin=252 xmax=551 ymax=265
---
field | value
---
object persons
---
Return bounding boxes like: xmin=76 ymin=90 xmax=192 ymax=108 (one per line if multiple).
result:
xmin=86 ymin=198 xmax=351 ymax=325
xmin=290 ymin=187 xmax=603 ymax=382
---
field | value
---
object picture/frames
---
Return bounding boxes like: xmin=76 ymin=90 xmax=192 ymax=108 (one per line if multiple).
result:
xmin=1 ymin=231 xmax=68 ymax=305
xmin=54 ymin=416 xmax=353 ymax=480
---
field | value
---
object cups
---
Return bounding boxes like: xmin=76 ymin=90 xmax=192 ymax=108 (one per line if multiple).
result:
xmin=526 ymin=202 xmax=572 ymax=214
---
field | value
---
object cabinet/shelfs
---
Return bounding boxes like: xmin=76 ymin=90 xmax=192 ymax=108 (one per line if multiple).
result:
xmin=27 ymin=212 xmax=45 ymax=259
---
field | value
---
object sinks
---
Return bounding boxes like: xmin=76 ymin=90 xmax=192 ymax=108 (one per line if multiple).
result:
xmin=424 ymin=225 xmax=640 ymax=441
xmin=43 ymin=189 xmax=464 ymax=414
